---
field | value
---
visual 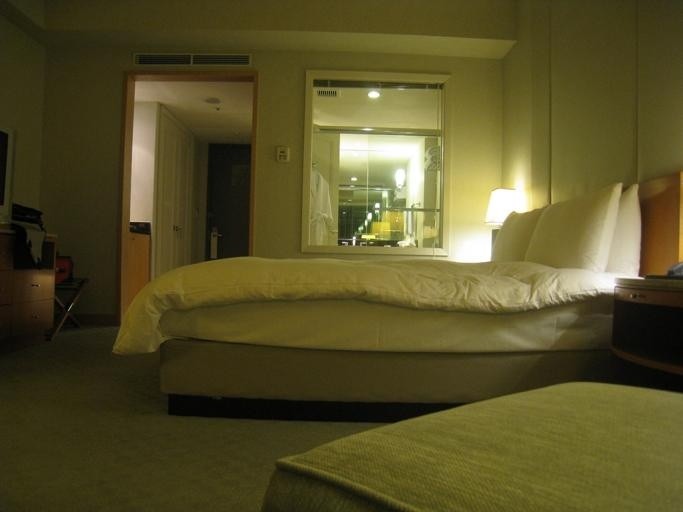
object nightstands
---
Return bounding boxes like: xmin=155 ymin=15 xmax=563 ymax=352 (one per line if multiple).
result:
xmin=607 ymin=275 xmax=682 ymax=392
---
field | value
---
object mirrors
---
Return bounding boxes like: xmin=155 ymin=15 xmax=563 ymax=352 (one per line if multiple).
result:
xmin=299 ymin=69 xmax=453 ymax=259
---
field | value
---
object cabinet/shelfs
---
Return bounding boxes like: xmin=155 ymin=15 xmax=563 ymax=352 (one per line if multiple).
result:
xmin=129 ymin=232 xmax=150 ymax=302
xmin=1 ymin=228 xmax=59 ymax=358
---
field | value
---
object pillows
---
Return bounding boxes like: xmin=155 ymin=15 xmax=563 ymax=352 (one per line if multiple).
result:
xmin=489 ymin=180 xmax=644 ymax=280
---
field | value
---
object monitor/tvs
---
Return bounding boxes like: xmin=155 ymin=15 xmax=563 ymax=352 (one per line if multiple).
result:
xmin=0 ymin=127 xmax=16 ymax=225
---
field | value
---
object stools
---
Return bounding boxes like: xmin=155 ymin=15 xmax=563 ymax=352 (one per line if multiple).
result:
xmin=262 ymin=382 xmax=683 ymax=510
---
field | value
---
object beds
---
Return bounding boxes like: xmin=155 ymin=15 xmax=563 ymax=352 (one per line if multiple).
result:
xmin=113 ymin=169 xmax=682 ymax=417
